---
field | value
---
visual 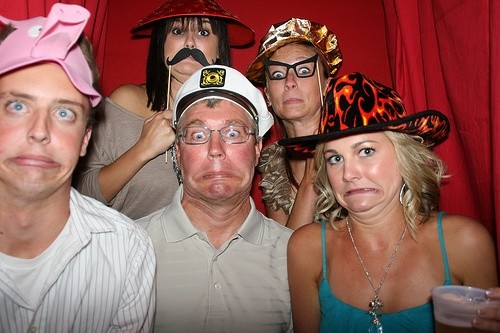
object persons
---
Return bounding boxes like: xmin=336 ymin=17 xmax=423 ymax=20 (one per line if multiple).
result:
xmin=0 ymin=3 xmax=156 ymax=333
xmin=77 ymin=0 xmax=254 ymax=220
xmin=134 ymin=65 xmax=294 ymax=332
xmin=247 ymin=17 xmax=343 ymax=231
xmin=278 ymin=72 xmax=500 ymax=333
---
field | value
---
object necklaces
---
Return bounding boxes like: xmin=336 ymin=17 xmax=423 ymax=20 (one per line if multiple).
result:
xmin=345 ymin=216 xmax=408 ymax=333
xmin=163 ymin=100 xmax=183 ymax=185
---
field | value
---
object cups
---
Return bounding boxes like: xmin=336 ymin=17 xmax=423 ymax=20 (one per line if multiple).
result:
xmin=431 ymin=284 xmax=491 ymax=333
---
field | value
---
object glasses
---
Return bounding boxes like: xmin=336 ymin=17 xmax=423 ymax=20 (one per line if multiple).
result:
xmin=178 ymin=124 xmax=258 ymax=145
xmin=262 ymin=54 xmax=319 ymax=80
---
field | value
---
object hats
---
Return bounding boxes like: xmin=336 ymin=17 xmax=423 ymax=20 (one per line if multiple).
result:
xmin=278 ymin=72 xmax=451 ymax=155
xmin=129 ymin=0 xmax=255 ymax=49
xmin=0 ymin=2 xmax=102 ymax=107
xmin=246 ymin=16 xmax=343 ymax=87
xmin=172 ymin=64 xmax=274 ymax=143
